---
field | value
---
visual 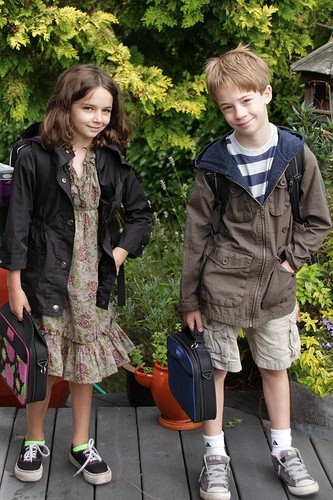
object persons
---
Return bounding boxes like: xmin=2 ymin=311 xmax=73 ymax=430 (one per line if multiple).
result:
xmin=179 ymin=45 xmax=332 ymax=500
xmin=0 ymin=64 xmax=155 ymax=485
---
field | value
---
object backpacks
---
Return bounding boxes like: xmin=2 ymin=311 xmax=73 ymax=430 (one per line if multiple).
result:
xmin=0 ymin=134 xmax=55 ymax=238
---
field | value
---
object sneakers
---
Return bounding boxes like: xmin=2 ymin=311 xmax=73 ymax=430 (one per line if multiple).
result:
xmin=69 ymin=438 xmax=112 ymax=485
xmin=271 ymin=448 xmax=319 ymax=496
xmin=198 ymin=453 xmax=231 ymax=500
xmin=14 ymin=438 xmax=50 ymax=482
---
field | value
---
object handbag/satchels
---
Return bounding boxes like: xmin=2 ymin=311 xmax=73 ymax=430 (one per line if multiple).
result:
xmin=0 ymin=300 xmax=49 ymax=404
xmin=167 ymin=321 xmax=217 ymax=423
